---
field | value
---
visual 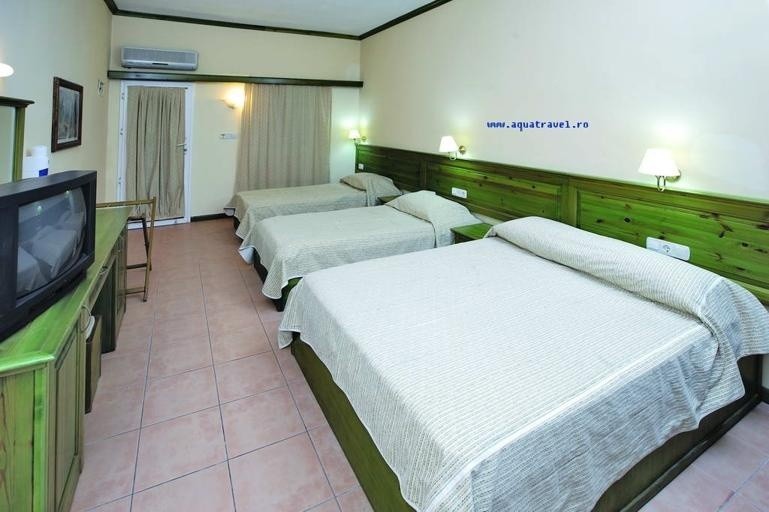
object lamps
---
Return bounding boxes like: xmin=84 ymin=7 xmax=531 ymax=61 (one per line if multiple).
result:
xmin=437 ymin=135 xmax=464 ymax=161
xmin=641 ymin=138 xmax=680 ymax=193
xmin=348 ymin=129 xmax=366 ymax=145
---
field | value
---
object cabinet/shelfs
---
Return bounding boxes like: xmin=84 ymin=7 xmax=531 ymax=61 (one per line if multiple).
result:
xmin=0 ymin=207 xmax=136 ymax=512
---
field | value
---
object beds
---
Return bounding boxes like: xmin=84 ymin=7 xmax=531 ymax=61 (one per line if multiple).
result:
xmin=236 ymin=190 xmax=478 ymax=310
xmin=222 ymin=171 xmax=397 ymax=240
xmin=287 ymin=215 xmax=769 ymax=512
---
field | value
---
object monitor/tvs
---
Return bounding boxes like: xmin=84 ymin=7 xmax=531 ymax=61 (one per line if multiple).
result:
xmin=0 ymin=170 xmax=97 ymax=344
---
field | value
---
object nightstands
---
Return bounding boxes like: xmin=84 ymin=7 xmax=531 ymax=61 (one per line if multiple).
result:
xmin=377 ymin=194 xmax=400 ymax=204
xmin=449 ymin=222 xmax=494 ymax=243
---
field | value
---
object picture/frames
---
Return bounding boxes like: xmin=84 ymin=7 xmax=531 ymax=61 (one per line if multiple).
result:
xmin=52 ymin=75 xmax=84 ymax=154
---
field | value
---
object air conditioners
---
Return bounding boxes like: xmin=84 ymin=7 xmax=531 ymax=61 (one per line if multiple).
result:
xmin=120 ymin=45 xmax=198 ymax=70
